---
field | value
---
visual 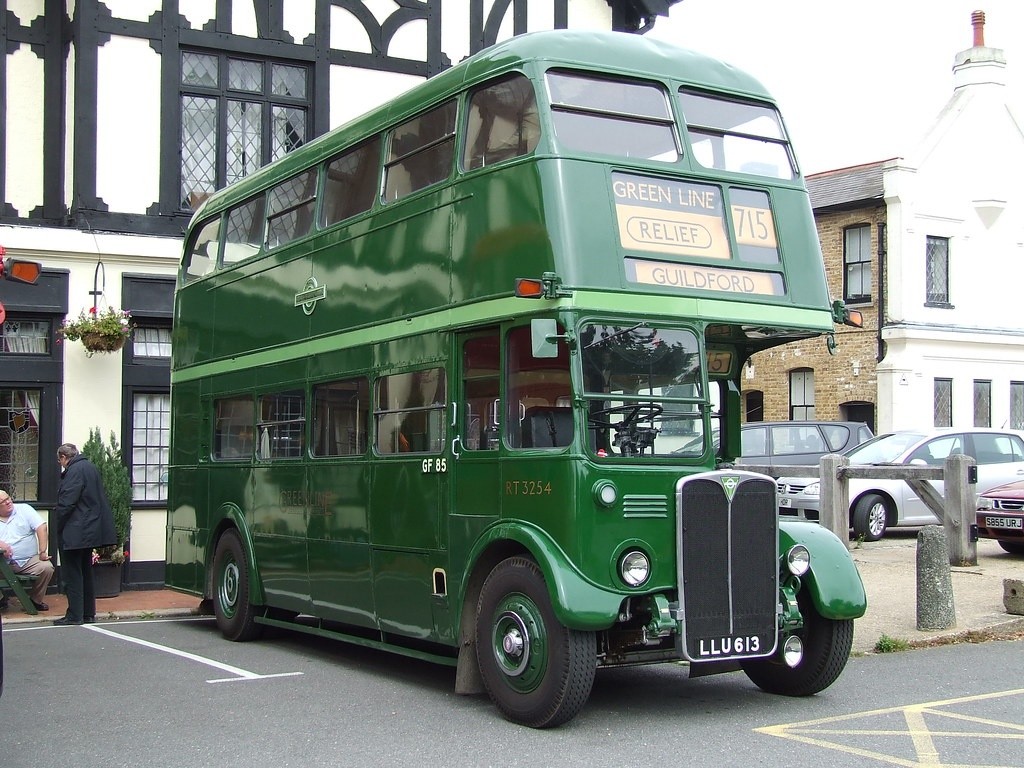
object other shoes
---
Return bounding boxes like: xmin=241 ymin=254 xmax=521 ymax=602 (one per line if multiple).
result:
xmin=30 ymin=597 xmax=49 ymax=611
xmin=84 ymin=616 xmax=96 ymax=623
xmin=0 ymin=597 xmax=8 ymax=609
xmin=53 ymin=616 xmax=83 ymax=624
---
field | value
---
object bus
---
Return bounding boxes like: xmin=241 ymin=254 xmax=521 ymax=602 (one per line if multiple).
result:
xmin=163 ymin=25 xmax=867 ymax=729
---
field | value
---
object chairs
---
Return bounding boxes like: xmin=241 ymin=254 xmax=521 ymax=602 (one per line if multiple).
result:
xmin=905 ymin=442 xmax=934 ymax=465
xmin=522 ymin=406 xmax=596 ymax=458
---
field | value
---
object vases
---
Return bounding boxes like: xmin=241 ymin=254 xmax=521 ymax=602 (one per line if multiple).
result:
xmin=79 ymin=331 xmax=127 ymax=351
xmin=91 ymin=560 xmax=122 ymax=598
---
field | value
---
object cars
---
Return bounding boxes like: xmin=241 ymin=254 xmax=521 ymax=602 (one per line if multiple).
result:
xmin=976 ymin=481 xmax=1024 ymax=552
xmin=671 ymin=420 xmax=887 ymax=465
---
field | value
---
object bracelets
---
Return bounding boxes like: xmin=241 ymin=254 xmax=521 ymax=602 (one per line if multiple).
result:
xmin=41 ymin=550 xmax=47 ymax=553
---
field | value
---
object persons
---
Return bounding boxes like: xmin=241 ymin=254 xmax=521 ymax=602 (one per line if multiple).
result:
xmin=0 ymin=489 xmax=55 ymax=611
xmin=48 ymin=442 xmax=119 ymax=625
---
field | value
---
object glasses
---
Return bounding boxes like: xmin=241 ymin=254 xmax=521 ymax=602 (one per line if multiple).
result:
xmin=57 ymin=455 xmax=63 ymax=463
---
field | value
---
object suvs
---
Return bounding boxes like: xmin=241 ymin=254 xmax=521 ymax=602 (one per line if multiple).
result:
xmin=775 ymin=428 xmax=1024 ymax=541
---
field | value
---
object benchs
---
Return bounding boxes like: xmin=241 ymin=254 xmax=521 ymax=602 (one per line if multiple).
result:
xmin=0 ymin=549 xmax=41 ymax=615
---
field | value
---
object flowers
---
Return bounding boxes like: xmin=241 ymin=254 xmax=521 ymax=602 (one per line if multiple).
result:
xmin=55 ymin=306 xmax=137 ymax=359
xmin=79 ymin=426 xmax=134 ymax=567
